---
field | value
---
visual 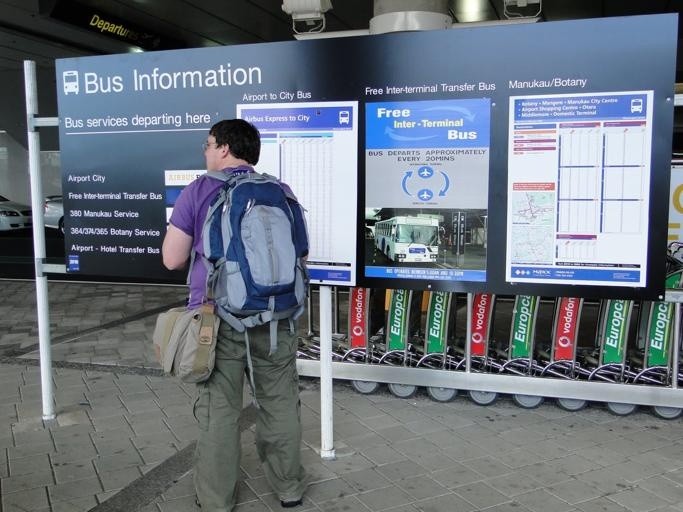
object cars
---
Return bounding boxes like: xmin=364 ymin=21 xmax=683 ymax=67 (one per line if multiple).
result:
xmin=0 ymin=195 xmax=32 ymax=231
xmin=44 ymin=194 xmax=65 ymax=235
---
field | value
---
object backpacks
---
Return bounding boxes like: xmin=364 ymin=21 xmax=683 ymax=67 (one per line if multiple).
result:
xmin=186 ymin=170 xmax=311 ymax=410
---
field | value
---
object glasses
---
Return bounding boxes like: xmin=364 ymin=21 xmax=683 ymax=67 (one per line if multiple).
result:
xmin=202 ymin=142 xmax=218 ymax=150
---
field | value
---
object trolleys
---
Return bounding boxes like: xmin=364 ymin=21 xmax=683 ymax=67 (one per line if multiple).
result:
xmin=298 ymin=242 xmax=683 ymax=421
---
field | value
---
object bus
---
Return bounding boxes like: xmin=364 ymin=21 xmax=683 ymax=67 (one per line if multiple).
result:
xmin=667 ymin=94 xmax=683 ymax=262
xmin=373 ymin=215 xmax=447 ymax=264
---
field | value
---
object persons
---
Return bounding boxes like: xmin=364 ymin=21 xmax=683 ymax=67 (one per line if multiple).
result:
xmin=159 ymin=118 xmax=312 ymax=511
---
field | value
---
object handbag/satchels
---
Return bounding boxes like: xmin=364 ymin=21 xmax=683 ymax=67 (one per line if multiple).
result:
xmin=152 ymin=303 xmax=220 ymax=384
